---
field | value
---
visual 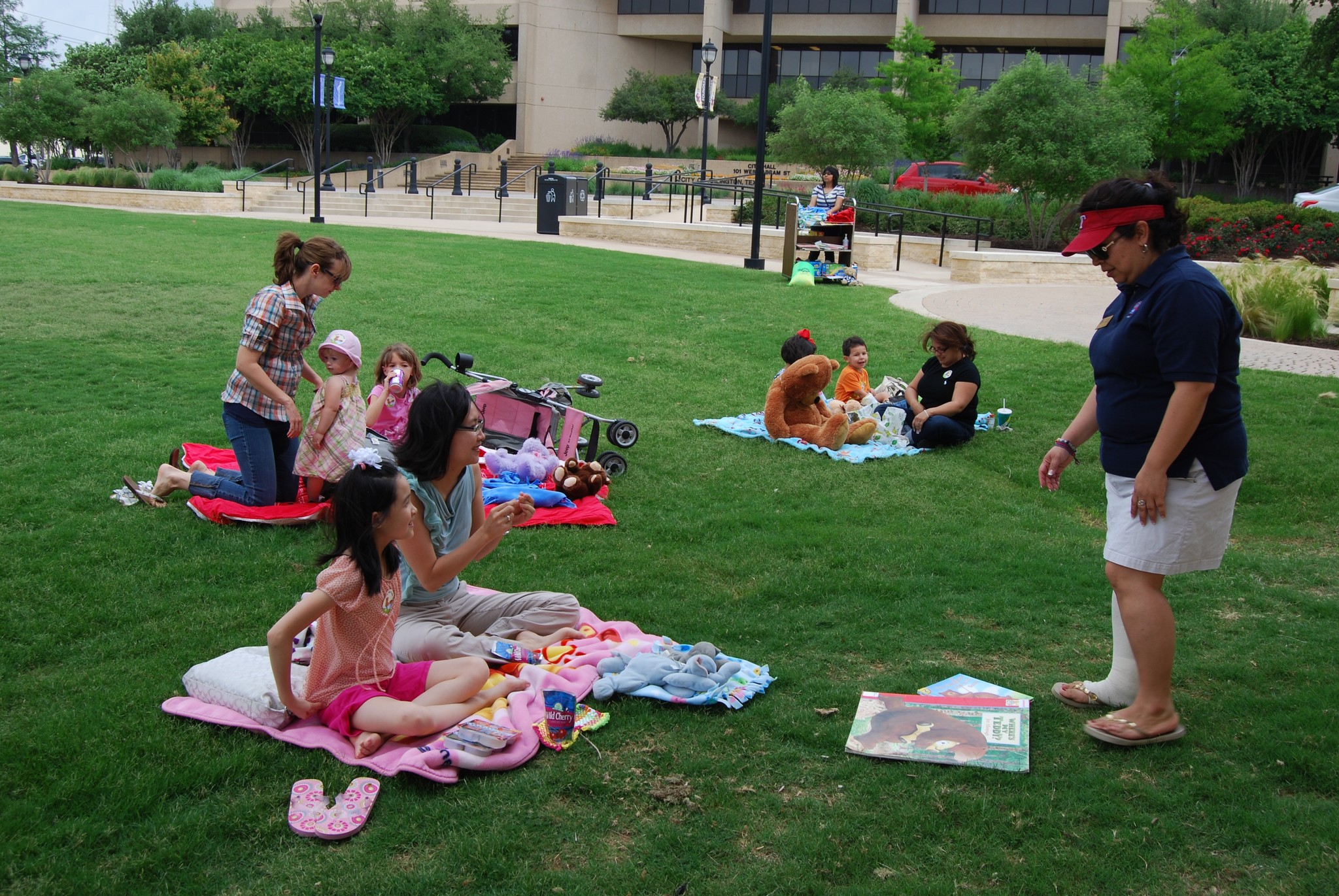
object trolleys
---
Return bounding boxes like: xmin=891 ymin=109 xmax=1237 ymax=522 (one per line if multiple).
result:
xmin=779 ymin=195 xmax=858 ymax=285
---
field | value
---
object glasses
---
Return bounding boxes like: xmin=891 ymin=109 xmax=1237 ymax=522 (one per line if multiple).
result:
xmin=929 ymin=342 xmax=954 ymax=353
xmin=456 ymin=419 xmax=485 ymax=436
xmin=1084 ymin=227 xmax=1135 ymax=260
xmin=308 ymin=261 xmax=343 ymax=284
xmin=823 ymin=172 xmax=832 ymax=175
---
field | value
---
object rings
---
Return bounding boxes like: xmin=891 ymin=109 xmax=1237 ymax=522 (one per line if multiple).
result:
xmin=1136 ymin=499 xmax=1145 ymax=505
xmin=506 ymin=515 xmax=511 ymax=522
xmin=917 ymin=422 xmax=920 ymax=424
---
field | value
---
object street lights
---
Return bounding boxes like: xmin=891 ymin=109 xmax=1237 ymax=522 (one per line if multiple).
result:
xmin=701 ymin=39 xmax=717 ymax=202
xmin=18 ymin=49 xmax=32 ymax=171
xmin=320 ymin=47 xmax=336 ymax=191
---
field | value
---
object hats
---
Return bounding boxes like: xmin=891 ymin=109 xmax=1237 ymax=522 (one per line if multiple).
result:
xmin=318 ymin=329 xmax=362 ymax=369
xmin=1060 ymin=205 xmax=1165 ymax=257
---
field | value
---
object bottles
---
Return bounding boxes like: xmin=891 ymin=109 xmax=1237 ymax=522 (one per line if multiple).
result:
xmin=842 ymin=234 xmax=849 ymax=249
xmin=389 ymin=368 xmax=404 ymax=393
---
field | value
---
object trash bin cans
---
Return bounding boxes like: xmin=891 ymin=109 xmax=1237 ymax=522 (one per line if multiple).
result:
xmin=537 ymin=173 xmax=577 ymax=235
xmin=571 ymin=175 xmax=588 ymax=217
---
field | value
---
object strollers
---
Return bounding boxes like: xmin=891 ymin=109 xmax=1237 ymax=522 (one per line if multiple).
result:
xmin=419 ymin=351 xmax=638 ymax=476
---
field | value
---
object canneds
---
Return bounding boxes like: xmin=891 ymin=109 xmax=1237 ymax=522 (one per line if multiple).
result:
xmin=389 ymin=368 xmax=404 ymax=392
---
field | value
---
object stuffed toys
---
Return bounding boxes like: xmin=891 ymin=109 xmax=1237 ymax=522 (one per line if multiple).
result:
xmin=484 ymin=436 xmax=558 ymax=482
xmin=592 ymin=641 xmax=744 ymax=700
xmin=554 ymin=458 xmax=611 ymax=500
xmin=764 ymin=354 xmax=876 ymax=450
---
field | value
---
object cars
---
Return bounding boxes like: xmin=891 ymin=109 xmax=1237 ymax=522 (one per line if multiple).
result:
xmin=893 ymin=162 xmax=1012 ymax=196
xmin=1293 ymin=182 xmax=1339 ymax=214
xmin=0 ymin=153 xmax=112 ymax=170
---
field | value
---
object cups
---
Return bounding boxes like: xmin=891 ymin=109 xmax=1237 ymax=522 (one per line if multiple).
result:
xmin=997 ymin=408 xmax=1012 ymax=429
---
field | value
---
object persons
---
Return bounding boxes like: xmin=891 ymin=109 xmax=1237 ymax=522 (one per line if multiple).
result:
xmin=381 ymin=378 xmax=586 ymax=667
xmin=899 ymin=320 xmax=982 ymax=449
xmin=804 ymin=166 xmax=846 ymax=281
xmin=365 ymin=343 xmax=421 ymax=451
xmin=121 ymin=232 xmax=352 ymax=509
xmin=772 ymin=329 xmax=892 ymax=413
xmin=264 ymin=449 xmax=532 ymax=758
xmin=1036 ymin=176 xmax=1250 ymax=746
xmin=291 ymin=330 xmax=366 ymax=502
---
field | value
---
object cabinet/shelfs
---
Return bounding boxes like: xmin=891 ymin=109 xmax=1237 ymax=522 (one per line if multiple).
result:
xmin=780 ymin=194 xmax=857 ymax=286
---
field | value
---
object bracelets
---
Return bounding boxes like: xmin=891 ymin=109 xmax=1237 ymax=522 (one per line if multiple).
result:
xmin=924 ymin=409 xmax=930 ymax=419
xmin=1054 ymin=437 xmax=1081 ymax=465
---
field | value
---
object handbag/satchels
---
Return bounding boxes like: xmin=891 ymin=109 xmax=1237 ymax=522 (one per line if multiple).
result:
xmin=182 ymin=646 xmax=311 ymax=730
xmin=888 ymin=390 xmax=911 ymax=409
xmin=875 ymin=375 xmax=909 ymax=397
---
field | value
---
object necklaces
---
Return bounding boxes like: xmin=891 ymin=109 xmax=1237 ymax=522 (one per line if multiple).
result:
xmin=380 ymin=559 xmax=388 ymax=580
xmin=289 ymin=276 xmax=297 ymax=295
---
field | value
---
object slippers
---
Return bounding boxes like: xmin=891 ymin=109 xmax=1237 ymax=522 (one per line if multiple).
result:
xmin=314 ymin=777 xmax=381 ymax=841
xmin=1051 ymin=682 xmax=1125 ymax=710
xmin=123 ymin=475 xmax=167 ymax=508
xmin=1082 ymin=711 xmax=1186 ymax=746
xmin=169 ymin=448 xmax=183 ymax=471
xmin=287 ymin=779 xmax=329 ymax=837
